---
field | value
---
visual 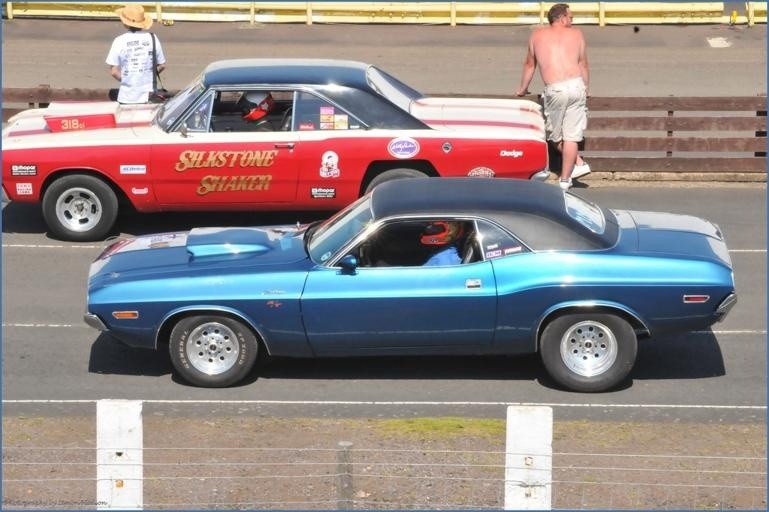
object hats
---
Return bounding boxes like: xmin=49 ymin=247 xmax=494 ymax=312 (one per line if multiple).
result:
xmin=119 ymin=4 xmax=153 ymax=30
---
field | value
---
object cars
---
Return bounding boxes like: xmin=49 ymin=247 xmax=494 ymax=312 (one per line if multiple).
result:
xmin=83 ymin=177 xmax=737 ymax=392
xmin=0 ymin=58 xmax=550 ymax=241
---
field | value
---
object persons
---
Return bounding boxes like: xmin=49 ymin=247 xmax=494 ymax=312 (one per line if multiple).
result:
xmin=516 ymin=4 xmax=591 ymax=190
xmin=235 ymin=91 xmax=275 ymax=131
xmin=105 ymin=3 xmax=166 ymax=105
xmin=419 ymin=220 xmax=465 ymax=265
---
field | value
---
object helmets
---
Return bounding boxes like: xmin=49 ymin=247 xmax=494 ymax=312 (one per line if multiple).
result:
xmin=421 ymin=221 xmax=459 ymax=244
xmin=237 ymin=91 xmax=274 ymax=122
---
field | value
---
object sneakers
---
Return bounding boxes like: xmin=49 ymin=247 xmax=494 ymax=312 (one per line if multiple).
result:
xmin=570 ymin=162 xmax=590 ymax=178
xmin=558 ymin=176 xmax=573 ymax=191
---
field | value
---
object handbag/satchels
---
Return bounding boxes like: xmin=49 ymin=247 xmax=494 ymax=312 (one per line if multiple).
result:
xmin=149 ymin=88 xmax=171 ymax=103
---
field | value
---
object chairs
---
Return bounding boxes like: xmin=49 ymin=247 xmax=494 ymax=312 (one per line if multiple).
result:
xmin=462 ymin=230 xmax=479 ymax=264
xmin=280 ymin=107 xmax=292 ymax=131
